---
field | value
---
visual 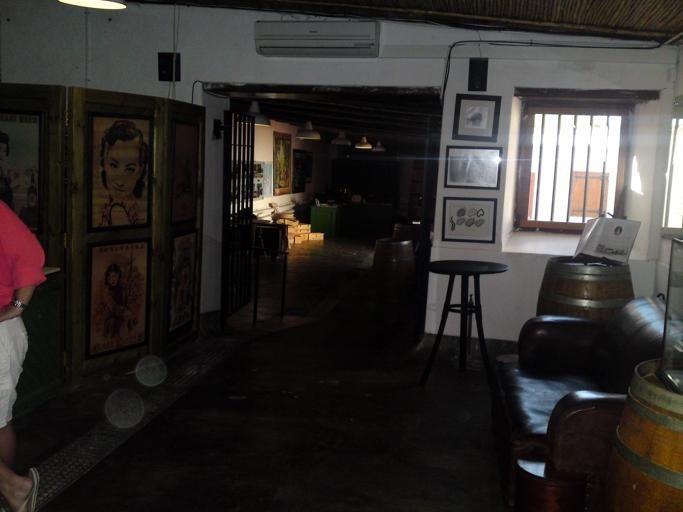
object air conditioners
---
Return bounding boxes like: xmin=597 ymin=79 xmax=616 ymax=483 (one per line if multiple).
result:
xmin=255 ymin=21 xmax=380 ymax=58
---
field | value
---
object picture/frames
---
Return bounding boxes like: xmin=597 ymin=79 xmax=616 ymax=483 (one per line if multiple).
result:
xmin=273 ymin=130 xmax=314 ymax=196
xmin=172 ymin=122 xmax=199 ymax=218
xmin=442 ymin=93 xmax=502 ymax=244
xmin=0 ymin=110 xmax=48 ymax=234
xmin=655 ymin=236 xmax=683 ymax=396
xmin=86 ymin=102 xmax=154 ymax=233
xmin=85 ymin=236 xmax=152 ymax=359
xmin=167 ymin=229 xmax=198 ymax=332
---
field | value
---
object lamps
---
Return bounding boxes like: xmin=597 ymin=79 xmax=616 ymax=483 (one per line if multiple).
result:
xmin=296 ymin=120 xmax=387 ymax=152
xmin=55 ymin=0 xmax=128 ymax=10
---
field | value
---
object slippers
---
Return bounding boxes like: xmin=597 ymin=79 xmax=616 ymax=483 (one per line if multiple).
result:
xmin=16 ymin=467 xmax=39 ymax=512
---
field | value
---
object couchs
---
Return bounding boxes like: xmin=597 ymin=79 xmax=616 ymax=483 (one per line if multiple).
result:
xmin=487 ymin=297 xmax=682 ymax=512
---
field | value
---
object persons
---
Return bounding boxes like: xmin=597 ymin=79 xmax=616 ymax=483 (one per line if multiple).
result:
xmin=100 ymin=121 xmax=147 ymax=224
xmin=103 ymin=263 xmax=134 ymax=340
xmin=0 ymin=201 xmax=47 ymax=512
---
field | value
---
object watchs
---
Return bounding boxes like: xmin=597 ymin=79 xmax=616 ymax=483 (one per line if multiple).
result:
xmin=13 ymin=299 xmax=27 ymax=310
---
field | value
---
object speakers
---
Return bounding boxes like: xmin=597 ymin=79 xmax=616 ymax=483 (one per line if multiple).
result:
xmin=468 ymin=57 xmax=488 ymax=91
xmin=158 ymin=52 xmax=180 ymax=81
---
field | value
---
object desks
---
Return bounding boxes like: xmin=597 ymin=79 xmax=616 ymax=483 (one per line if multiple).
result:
xmin=419 ymin=260 xmax=508 ymax=387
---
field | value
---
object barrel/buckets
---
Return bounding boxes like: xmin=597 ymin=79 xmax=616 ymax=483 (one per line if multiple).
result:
xmin=536 ymin=253 xmax=637 ymax=324
xmin=603 ymin=356 xmax=683 ymax=511
xmin=375 ymin=235 xmax=417 ymax=326
xmin=408 ymin=172 xmax=434 ymax=238
